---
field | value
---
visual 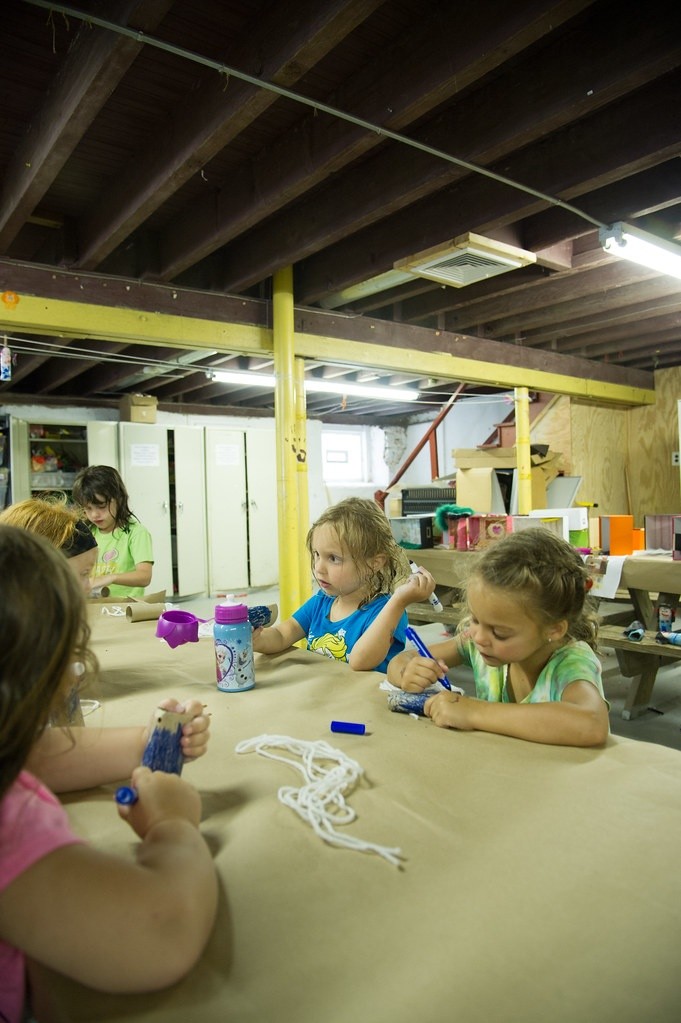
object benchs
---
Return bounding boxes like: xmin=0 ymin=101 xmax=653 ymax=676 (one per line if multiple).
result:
xmin=404 ymin=602 xmax=681 ymax=723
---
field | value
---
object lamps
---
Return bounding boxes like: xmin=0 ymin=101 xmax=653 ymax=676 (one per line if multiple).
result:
xmin=597 ymin=222 xmax=680 ymax=282
xmin=204 ymin=368 xmax=422 ymax=402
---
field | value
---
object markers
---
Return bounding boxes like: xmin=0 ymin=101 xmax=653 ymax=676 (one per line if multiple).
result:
xmin=404 ymin=626 xmax=453 ymax=692
xmin=114 ymin=786 xmax=139 ymax=807
xmin=408 ymin=558 xmax=444 ymax=613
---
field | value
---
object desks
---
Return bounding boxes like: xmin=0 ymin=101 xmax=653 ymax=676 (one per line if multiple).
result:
xmin=23 ymin=600 xmax=680 ymax=1023
xmin=400 ymin=543 xmax=680 ymax=632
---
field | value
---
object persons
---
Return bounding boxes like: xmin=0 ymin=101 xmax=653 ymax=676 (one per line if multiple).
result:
xmin=383 ymin=525 xmax=614 ymax=748
xmin=67 ymin=464 xmax=157 ymax=596
xmin=1 ymin=492 xmax=99 ymax=606
xmin=0 ymin=519 xmax=223 ymax=1023
xmin=248 ymin=491 xmax=438 ymax=675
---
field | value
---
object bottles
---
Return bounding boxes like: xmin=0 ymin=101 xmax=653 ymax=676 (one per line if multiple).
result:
xmin=213 ymin=593 xmax=255 ymax=693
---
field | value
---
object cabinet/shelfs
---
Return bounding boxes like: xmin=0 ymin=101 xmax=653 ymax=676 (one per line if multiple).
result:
xmin=8 ymin=415 xmax=122 ymax=509
xmin=118 ymin=422 xmax=210 ymax=604
xmin=204 ymin=426 xmax=279 ymax=596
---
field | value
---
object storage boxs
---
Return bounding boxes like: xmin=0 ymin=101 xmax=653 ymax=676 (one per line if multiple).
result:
xmin=119 ymin=392 xmax=159 ymax=424
xmin=388 ymin=515 xmax=434 ymax=550
